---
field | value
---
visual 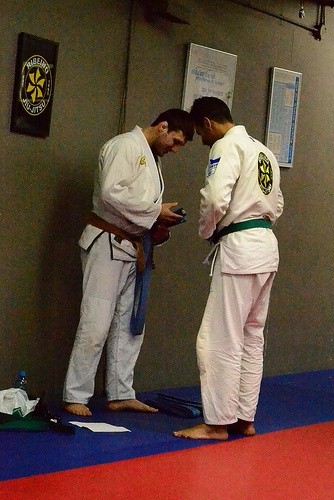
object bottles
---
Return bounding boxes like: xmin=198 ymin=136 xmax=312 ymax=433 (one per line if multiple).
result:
xmin=28 ymin=396 xmax=61 ymax=425
xmin=14 ymin=371 xmax=27 ymax=392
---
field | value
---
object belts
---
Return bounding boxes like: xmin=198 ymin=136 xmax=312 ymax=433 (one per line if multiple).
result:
xmin=87 ymin=213 xmax=148 ymax=271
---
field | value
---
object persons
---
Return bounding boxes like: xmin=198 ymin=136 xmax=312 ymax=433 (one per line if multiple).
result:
xmin=61 ymin=107 xmax=196 ymax=416
xmin=173 ymin=95 xmax=285 ymax=441
